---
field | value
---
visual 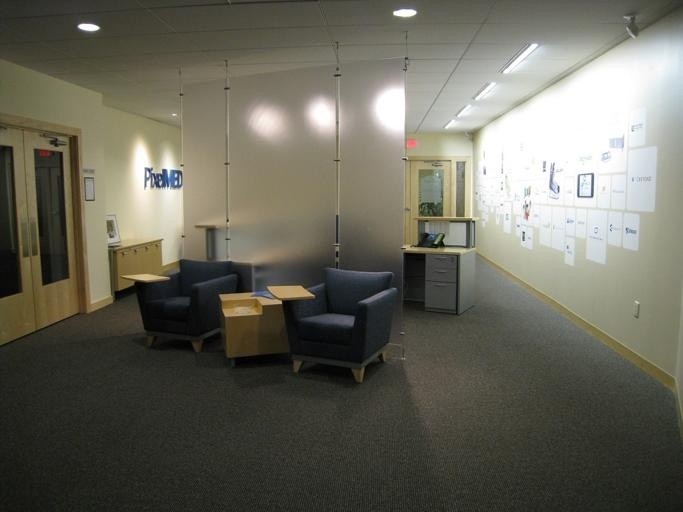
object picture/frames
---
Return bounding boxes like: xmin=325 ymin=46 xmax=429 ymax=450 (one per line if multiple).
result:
xmin=106 ymin=214 xmax=121 ymax=245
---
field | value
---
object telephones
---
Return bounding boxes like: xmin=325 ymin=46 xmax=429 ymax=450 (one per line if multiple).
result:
xmin=416 ymin=232 xmax=445 ymax=248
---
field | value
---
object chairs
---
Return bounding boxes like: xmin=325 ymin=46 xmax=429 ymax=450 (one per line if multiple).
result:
xmin=134 ymin=260 xmax=398 ymax=383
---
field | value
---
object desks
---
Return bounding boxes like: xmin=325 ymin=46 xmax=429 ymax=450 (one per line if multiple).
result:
xmin=404 ymin=246 xmax=477 ymax=316
xmin=108 ymin=236 xmax=164 ymax=301
